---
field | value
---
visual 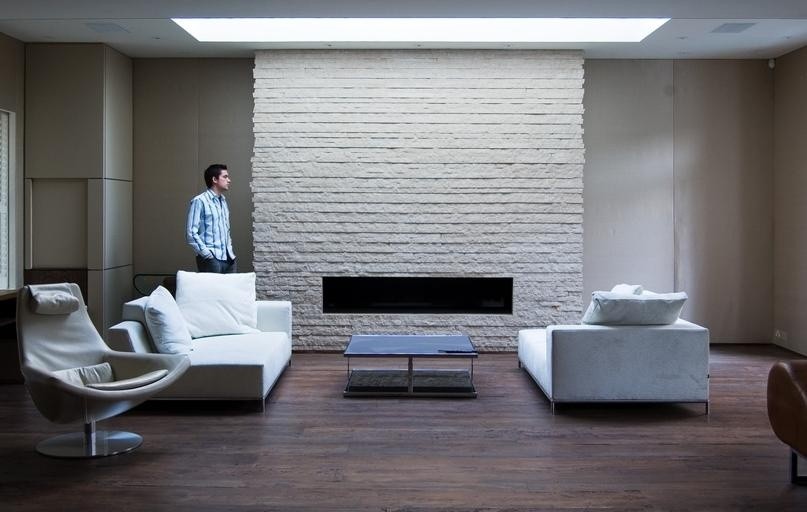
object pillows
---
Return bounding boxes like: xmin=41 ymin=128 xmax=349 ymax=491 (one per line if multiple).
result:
xmin=582 ymin=291 xmax=689 ymax=326
xmin=145 ymin=285 xmax=193 ymax=358
xmin=175 ymin=270 xmax=257 ymax=339
xmin=610 ymin=283 xmax=643 ymax=295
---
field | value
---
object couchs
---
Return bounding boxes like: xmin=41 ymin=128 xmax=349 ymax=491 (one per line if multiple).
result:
xmin=107 ymin=295 xmax=293 ymax=414
xmin=517 ymin=284 xmax=711 ymax=415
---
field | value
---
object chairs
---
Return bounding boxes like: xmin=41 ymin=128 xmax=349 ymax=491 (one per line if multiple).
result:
xmin=767 ymin=359 xmax=806 ymax=487
xmin=14 ymin=283 xmax=192 ymax=460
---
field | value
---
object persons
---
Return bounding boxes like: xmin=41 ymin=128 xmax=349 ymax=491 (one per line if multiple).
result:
xmin=184 ymin=164 xmax=238 ymax=274
